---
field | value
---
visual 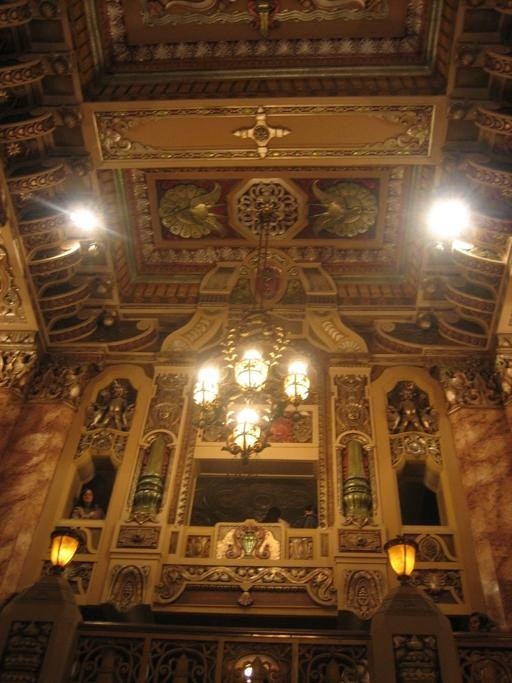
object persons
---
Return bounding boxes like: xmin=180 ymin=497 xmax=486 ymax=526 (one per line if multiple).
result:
xmin=97 ymin=387 xmax=127 ymax=429
xmin=263 ymin=508 xmax=291 ymax=529
xmin=70 ymin=488 xmax=105 ymax=518
xmin=302 ymin=504 xmax=317 ymax=527
xmin=398 ymin=388 xmax=425 ymax=433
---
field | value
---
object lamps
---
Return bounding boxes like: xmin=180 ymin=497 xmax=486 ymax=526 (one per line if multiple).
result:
xmin=383 ymin=534 xmax=419 ymax=586
xmin=193 ymin=212 xmax=308 ymax=467
xmin=50 ymin=524 xmax=87 ymax=574
